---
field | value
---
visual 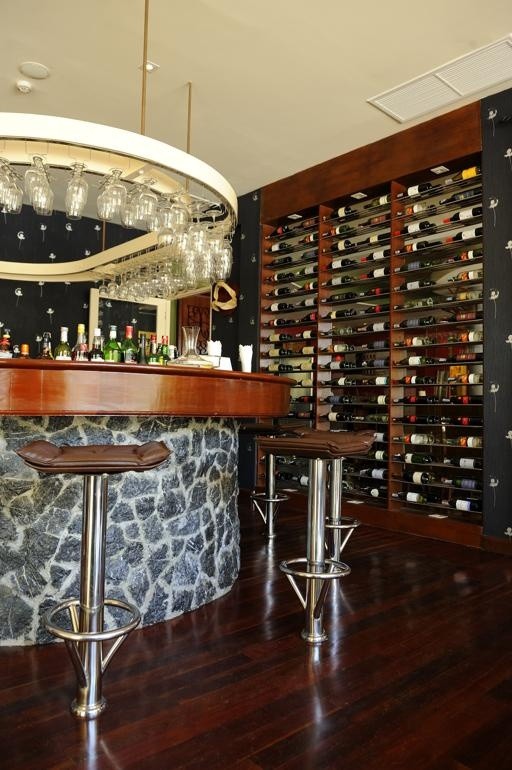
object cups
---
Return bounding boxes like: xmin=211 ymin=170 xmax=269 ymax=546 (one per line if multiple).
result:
xmin=207 ymin=344 xmax=221 ymax=356
xmin=168 ymin=345 xmax=177 ymax=358
xmin=239 ymin=351 xmax=253 ymax=372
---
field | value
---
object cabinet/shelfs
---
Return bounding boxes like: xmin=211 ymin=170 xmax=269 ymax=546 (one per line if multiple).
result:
xmin=257 ymin=172 xmax=484 ymax=517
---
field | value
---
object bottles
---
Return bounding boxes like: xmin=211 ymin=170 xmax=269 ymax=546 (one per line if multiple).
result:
xmin=260 ymin=215 xmax=318 ymax=419
xmin=37 ymin=331 xmax=53 ymax=360
xmin=72 ymin=324 xmax=170 ymax=365
xmin=168 ymin=325 xmax=214 ymax=368
xmin=1 ymin=327 xmax=30 ymax=358
xmin=291 ymin=473 xmax=310 ymax=487
xmin=360 ymin=430 xmax=388 ymax=497
xmin=319 ymin=193 xmax=392 ymax=425
xmin=390 ymin=164 xmax=482 ymax=511
xmin=54 ymin=326 xmax=71 ymax=361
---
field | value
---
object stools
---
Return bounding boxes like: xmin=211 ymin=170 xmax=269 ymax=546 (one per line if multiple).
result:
xmin=13 ymin=440 xmax=174 ymax=725
xmin=252 ymin=427 xmax=377 ymax=645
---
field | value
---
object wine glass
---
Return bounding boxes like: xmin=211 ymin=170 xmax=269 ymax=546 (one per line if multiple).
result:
xmin=0 ymin=155 xmax=235 ymax=302
